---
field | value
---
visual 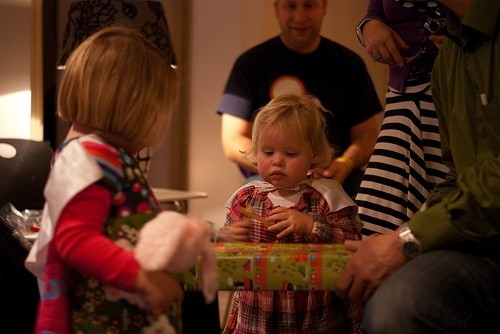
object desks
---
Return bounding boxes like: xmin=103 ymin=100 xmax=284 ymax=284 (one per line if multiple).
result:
xmin=150 ymin=188 xmax=209 ymax=213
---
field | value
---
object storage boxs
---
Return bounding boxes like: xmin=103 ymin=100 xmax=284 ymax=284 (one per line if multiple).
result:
xmin=182 ymin=242 xmax=355 ymax=291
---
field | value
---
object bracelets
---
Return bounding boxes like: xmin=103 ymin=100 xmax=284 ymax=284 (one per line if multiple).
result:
xmin=337 ymin=157 xmax=354 ymax=170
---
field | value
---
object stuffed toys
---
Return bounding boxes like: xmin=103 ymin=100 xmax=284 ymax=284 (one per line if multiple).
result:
xmin=103 ymin=209 xmax=222 ymax=307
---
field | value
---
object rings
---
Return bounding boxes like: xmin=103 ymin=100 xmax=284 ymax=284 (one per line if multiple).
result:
xmin=373 ymin=54 xmax=381 ymax=61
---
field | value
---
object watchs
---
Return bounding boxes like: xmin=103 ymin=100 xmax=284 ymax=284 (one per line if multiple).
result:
xmin=396 ymin=224 xmax=423 ymax=260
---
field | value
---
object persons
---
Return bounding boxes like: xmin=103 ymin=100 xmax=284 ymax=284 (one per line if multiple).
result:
xmin=22 ymin=26 xmax=183 ymax=334
xmin=355 ymin=0 xmax=453 ymax=240
xmin=216 ymin=0 xmax=385 ymax=199
xmin=335 ymin=0 xmax=500 ymax=334
xmin=215 ymin=93 xmax=368 ymax=334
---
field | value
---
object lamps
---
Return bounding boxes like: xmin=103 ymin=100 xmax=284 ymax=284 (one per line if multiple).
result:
xmin=57 ymin=0 xmax=178 ymax=70
